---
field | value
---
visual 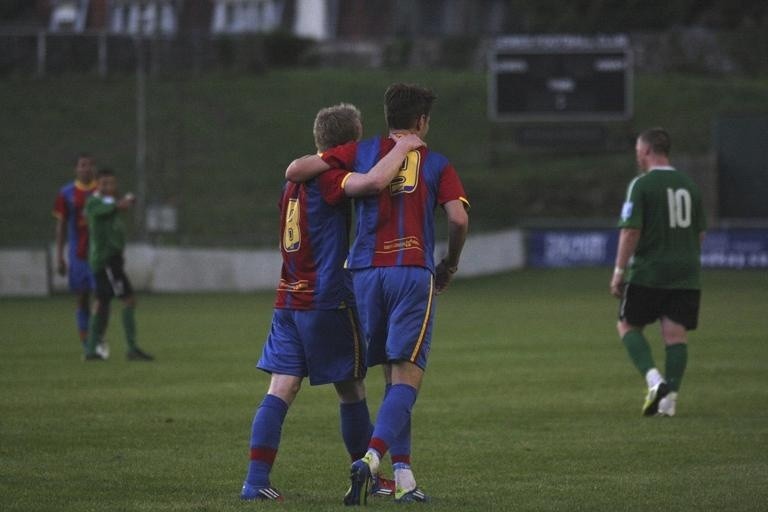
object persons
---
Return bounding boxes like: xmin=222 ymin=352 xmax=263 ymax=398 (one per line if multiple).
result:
xmin=86 ymin=167 xmax=157 ymax=361
xmin=285 ymin=80 xmax=472 ymax=506
xmin=240 ymin=101 xmax=428 ymax=506
xmin=609 ymin=127 xmax=708 ymax=417
xmin=52 ymin=154 xmax=115 ymax=359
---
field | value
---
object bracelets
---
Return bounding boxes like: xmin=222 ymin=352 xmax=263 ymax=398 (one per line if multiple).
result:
xmin=614 ymin=267 xmax=624 ymax=275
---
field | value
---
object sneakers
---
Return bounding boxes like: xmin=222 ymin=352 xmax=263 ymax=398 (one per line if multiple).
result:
xmin=343 ymin=457 xmax=375 ymax=506
xmin=640 ymin=377 xmax=677 ymax=417
xmin=239 ymin=481 xmax=285 ymax=503
xmin=83 ymin=341 xmax=155 ymax=362
xmin=372 ymin=476 xmax=396 ymax=499
xmin=394 ymin=483 xmax=435 ymax=504
